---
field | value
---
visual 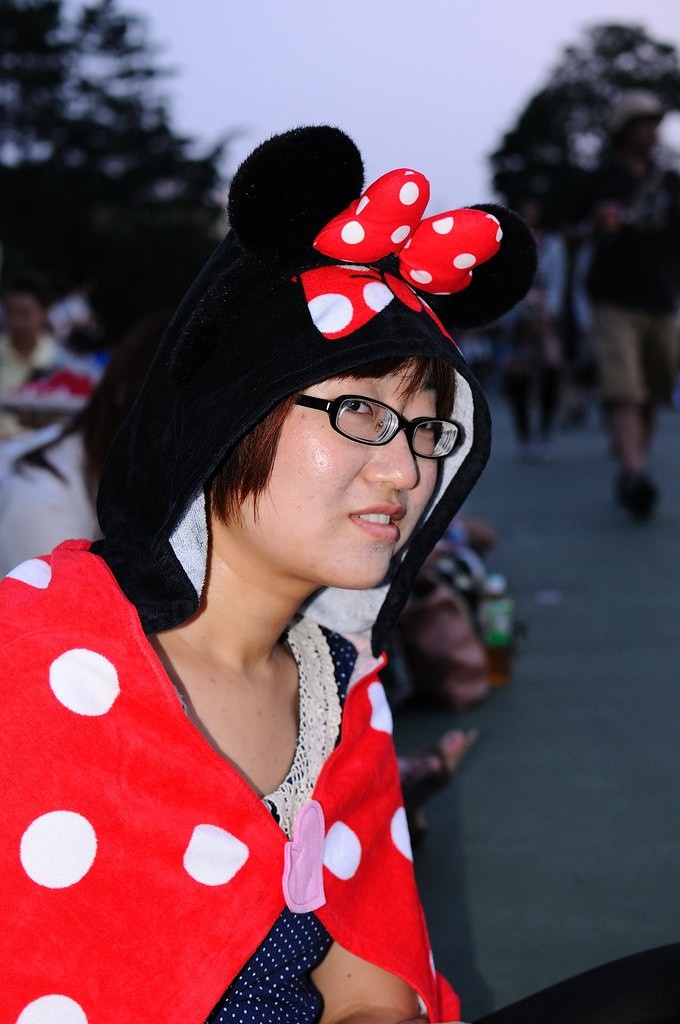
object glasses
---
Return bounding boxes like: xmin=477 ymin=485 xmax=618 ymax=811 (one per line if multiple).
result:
xmin=294 ymin=394 xmax=466 ymax=459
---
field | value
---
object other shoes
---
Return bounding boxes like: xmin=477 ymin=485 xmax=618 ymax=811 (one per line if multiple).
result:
xmin=614 ymin=471 xmax=657 ymax=520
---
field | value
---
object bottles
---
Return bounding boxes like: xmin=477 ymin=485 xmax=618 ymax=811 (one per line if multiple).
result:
xmin=479 ymin=573 xmax=515 ymax=675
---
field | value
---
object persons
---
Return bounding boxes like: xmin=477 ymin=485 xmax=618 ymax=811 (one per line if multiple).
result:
xmin=1 ymin=87 xmax=680 ymax=857
xmin=0 ymin=123 xmax=536 ymax=1024
